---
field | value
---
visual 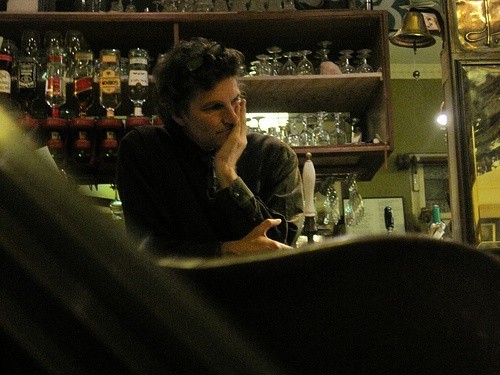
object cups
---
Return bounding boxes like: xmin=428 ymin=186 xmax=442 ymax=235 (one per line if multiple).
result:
xmin=37 ymin=0 xmax=299 ymax=13
xmin=21 ymin=30 xmax=83 ymax=55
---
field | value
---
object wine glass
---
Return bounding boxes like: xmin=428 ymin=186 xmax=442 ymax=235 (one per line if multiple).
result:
xmin=242 ymin=110 xmax=362 ymax=147
xmin=237 ymin=40 xmax=373 ymax=77
xmin=109 ymin=185 xmax=124 ymax=214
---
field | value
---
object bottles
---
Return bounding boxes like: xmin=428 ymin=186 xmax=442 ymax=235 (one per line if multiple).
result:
xmin=0 ymin=34 xmax=14 ymax=103
xmin=419 ymin=204 xmax=452 ymax=241
xmin=0 ymin=48 xmax=173 ymax=180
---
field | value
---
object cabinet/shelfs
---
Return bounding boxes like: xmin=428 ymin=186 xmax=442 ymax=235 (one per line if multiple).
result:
xmin=0 ymin=10 xmax=391 ymax=185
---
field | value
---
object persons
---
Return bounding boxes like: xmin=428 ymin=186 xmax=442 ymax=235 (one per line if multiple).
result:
xmin=118 ymin=39 xmax=306 ymax=259
